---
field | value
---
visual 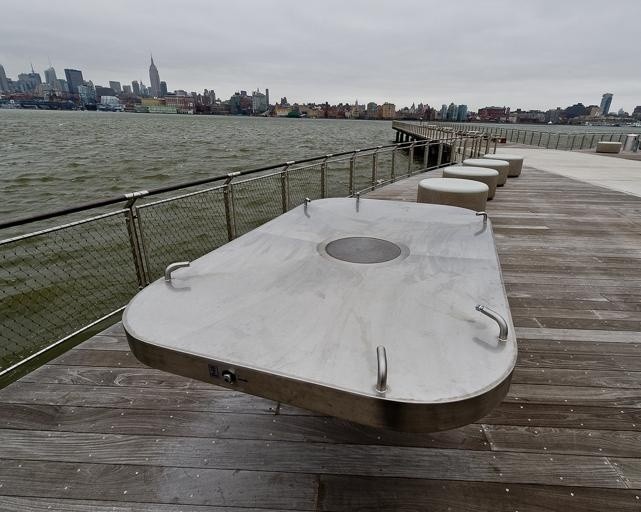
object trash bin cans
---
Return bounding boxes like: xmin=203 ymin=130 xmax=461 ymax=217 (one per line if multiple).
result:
xmin=624 ymin=134 xmax=640 ymax=152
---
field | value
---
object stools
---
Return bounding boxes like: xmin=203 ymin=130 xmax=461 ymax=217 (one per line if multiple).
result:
xmin=416 ymin=152 xmax=525 ymax=213
xmin=596 ymin=140 xmax=623 ymax=154
